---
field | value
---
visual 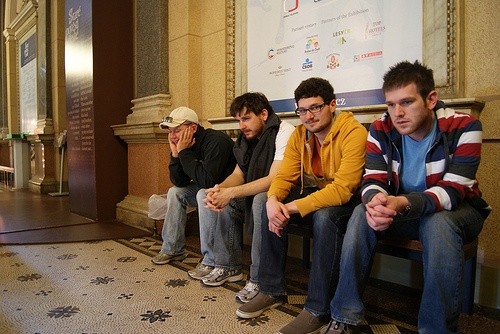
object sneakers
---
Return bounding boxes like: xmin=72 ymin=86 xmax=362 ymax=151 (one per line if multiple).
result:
xmin=151 ymin=250 xmax=189 ymax=265
xmin=188 ymin=262 xmax=214 ymax=280
xmin=200 ymin=267 xmax=244 ymax=287
xmin=235 ymin=280 xmax=259 ymax=302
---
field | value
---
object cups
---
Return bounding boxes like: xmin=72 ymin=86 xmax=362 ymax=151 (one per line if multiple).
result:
xmin=0 ymin=127 xmax=8 ymax=140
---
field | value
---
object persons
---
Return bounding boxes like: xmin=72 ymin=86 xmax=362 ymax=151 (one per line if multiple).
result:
xmin=323 ymin=60 xmax=492 ymax=334
xmin=151 ymin=106 xmax=237 ymax=280
xmin=235 ymin=77 xmax=369 ymax=334
xmin=201 ymin=91 xmax=295 ymax=304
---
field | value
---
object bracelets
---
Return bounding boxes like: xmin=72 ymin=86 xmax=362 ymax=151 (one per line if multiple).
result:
xmin=398 ymin=205 xmax=411 ymax=216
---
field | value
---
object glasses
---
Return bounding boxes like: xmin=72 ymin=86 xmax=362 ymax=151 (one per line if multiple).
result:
xmin=295 ymin=102 xmax=325 ymax=116
xmin=161 ymin=116 xmax=176 ymax=123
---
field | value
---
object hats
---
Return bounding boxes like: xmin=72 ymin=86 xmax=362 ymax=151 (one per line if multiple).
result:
xmin=159 ymin=106 xmax=198 ymax=129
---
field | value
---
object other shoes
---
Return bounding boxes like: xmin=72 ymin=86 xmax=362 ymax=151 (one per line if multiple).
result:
xmin=276 ymin=308 xmax=331 ymax=334
xmin=235 ymin=292 xmax=287 ymax=319
xmin=322 ymin=318 xmax=356 ymax=334
